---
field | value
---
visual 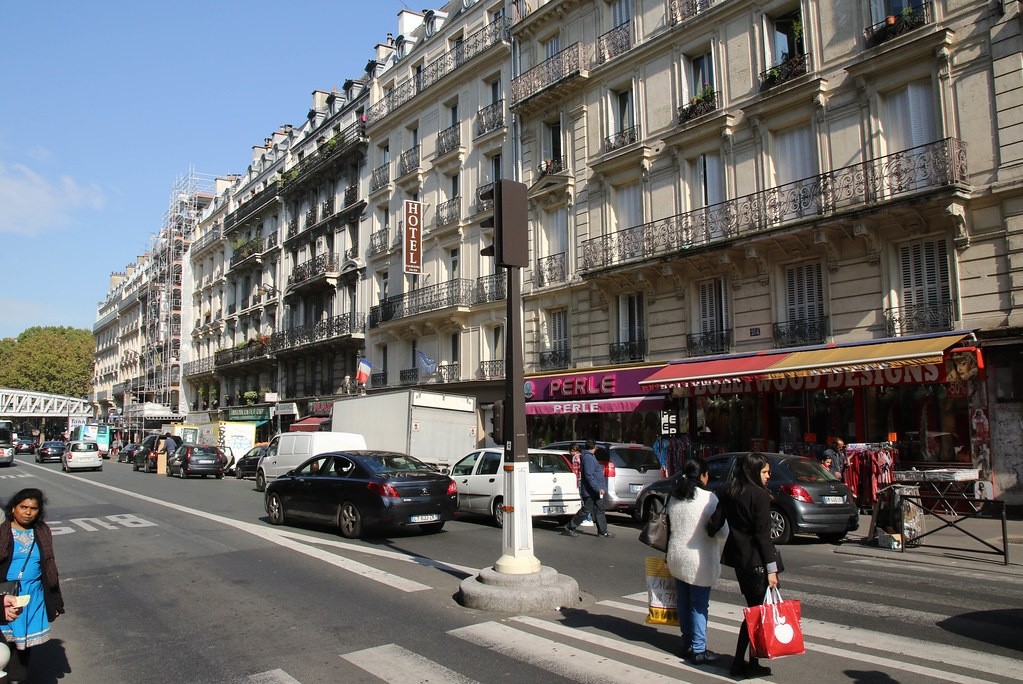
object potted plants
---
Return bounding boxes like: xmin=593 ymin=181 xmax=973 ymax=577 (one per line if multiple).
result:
xmin=243 ymin=391 xmax=257 ymax=405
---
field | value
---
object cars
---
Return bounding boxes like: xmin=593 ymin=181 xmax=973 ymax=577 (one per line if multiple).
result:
xmin=15 ymin=440 xmax=35 ymax=455
xmin=62 ymin=440 xmax=103 ymax=473
xmin=13 ymin=438 xmax=20 ymax=447
xmin=263 ymin=449 xmax=458 ymax=540
xmin=118 ymin=443 xmax=137 ymax=463
xmin=36 ymin=441 xmax=65 ymax=463
xmin=634 ymin=451 xmax=859 ymax=546
xmin=235 ymin=441 xmax=271 ymax=480
xmin=448 ymin=446 xmax=583 ymax=529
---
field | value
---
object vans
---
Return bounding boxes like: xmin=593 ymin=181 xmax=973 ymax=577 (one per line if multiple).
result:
xmin=255 ymin=430 xmax=369 ymax=493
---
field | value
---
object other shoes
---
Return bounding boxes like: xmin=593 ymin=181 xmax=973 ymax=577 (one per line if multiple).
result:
xmin=730 ymin=662 xmax=744 ymax=675
xmin=692 ymin=650 xmax=721 ymax=665
xmin=744 ymin=664 xmax=771 ymax=677
xmin=680 ymin=646 xmax=692 ymax=657
xmin=564 ymin=524 xmax=578 ymax=536
xmin=598 ymin=531 xmax=615 ymax=538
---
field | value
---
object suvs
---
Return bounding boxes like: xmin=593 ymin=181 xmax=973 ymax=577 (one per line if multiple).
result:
xmin=132 ymin=434 xmax=183 ymax=474
xmin=167 ymin=442 xmax=234 ymax=480
xmin=537 ymin=440 xmax=669 ymax=521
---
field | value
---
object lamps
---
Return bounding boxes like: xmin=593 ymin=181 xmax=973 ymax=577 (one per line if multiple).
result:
xmin=257 ymin=283 xmax=282 ymax=296
xmin=124 ymin=348 xmax=140 ymax=356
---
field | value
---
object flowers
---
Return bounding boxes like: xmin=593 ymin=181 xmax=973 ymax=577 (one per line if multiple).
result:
xmin=691 ymin=81 xmax=714 ymax=106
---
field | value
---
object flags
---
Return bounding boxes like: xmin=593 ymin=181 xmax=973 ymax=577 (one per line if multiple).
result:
xmin=418 ymin=351 xmax=437 ymax=375
xmin=355 ymin=358 xmax=372 ymax=384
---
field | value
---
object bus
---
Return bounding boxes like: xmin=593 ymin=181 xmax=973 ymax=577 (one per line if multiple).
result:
xmin=74 ymin=423 xmax=110 ymax=459
xmin=0 ymin=420 xmax=13 ymax=467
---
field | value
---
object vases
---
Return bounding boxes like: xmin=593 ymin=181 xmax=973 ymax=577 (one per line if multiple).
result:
xmin=884 ymin=16 xmax=895 ymax=26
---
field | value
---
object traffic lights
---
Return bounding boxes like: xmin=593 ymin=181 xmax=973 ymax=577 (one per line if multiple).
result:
xmin=480 ymin=177 xmax=529 ymax=269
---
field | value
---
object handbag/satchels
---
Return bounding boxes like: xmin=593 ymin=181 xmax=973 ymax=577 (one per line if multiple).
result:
xmin=638 ymin=494 xmax=671 ymax=552
xmin=743 ymin=585 xmax=806 ymax=660
xmin=646 ymin=553 xmax=680 ymax=626
xmin=0 ymin=580 xmax=23 ymax=621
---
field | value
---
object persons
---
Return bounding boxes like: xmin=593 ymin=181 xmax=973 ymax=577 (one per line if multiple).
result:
xmin=310 ymin=462 xmax=319 ymax=475
xmin=563 ymin=440 xmax=616 ymax=538
xmin=972 ymin=444 xmax=988 ymax=481
xmin=160 ymin=432 xmax=177 ymax=460
xmin=951 ymin=351 xmax=977 ymax=380
xmin=975 ymin=482 xmax=989 ymax=499
xmin=220 ymin=451 xmax=228 ymax=480
xmin=569 ymin=442 xmax=594 ymax=526
xmin=705 ymin=453 xmax=784 ymax=676
xmin=0 ymin=488 xmax=60 ymax=680
xmin=973 ymin=411 xmax=986 ymax=440
xmin=665 ymin=457 xmax=730 ymax=664
xmin=820 ymin=455 xmax=832 ymax=471
xmin=824 ymin=437 xmax=848 ymax=485
xmin=0 ymin=595 xmax=25 ymax=626
xmin=268 ymin=435 xmax=278 ymax=456
xmin=111 ymin=438 xmax=118 ymax=455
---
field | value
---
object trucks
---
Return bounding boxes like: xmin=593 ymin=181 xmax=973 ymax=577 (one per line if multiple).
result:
xmin=161 ymin=420 xmax=257 ymax=476
xmin=319 ymin=388 xmax=475 ymax=474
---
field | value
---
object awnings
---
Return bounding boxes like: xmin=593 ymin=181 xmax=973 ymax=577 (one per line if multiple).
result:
xmin=638 ymin=333 xmax=971 ymax=391
